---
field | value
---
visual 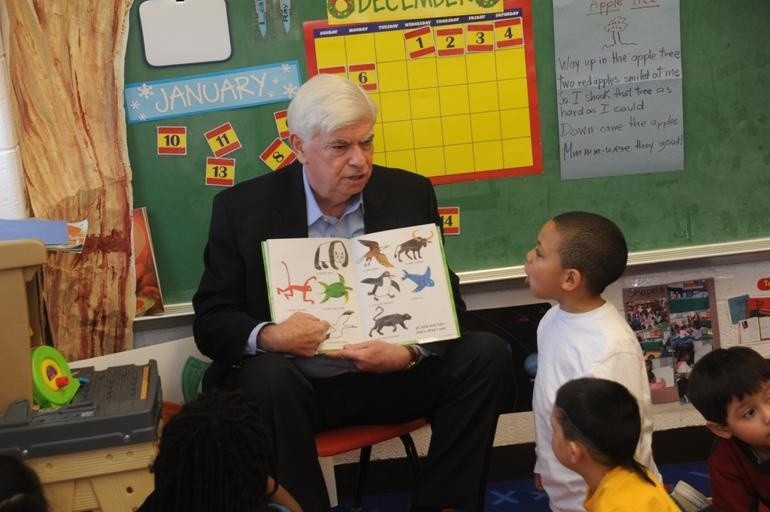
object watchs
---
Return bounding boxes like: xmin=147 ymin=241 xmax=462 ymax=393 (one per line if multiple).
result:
xmin=402 ymin=344 xmax=417 ymax=370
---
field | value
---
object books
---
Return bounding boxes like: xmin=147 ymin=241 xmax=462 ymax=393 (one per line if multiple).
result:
xmin=0 ymin=218 xmax=89 ymax=254
xmin=261 ymin=223 xmax=461 ymax=355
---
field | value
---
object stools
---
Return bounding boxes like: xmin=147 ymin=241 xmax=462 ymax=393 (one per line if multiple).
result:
xmin=312 ymin=421 xmax=432 ymax=509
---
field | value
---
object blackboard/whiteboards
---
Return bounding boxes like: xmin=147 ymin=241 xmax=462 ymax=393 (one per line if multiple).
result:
xmin=111 ymin=0 xmax=769 ymax=317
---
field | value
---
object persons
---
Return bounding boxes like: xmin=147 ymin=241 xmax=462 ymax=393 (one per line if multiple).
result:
xmin=550 ymin=377 xmax=683 ymax=512
xmin=193 ymin=73 xmax=513 ymax=512
xmin=524 ymin=211 xmax=664 ymax=512
xmin=685 ymin=345 xmax=770 ymax=512
